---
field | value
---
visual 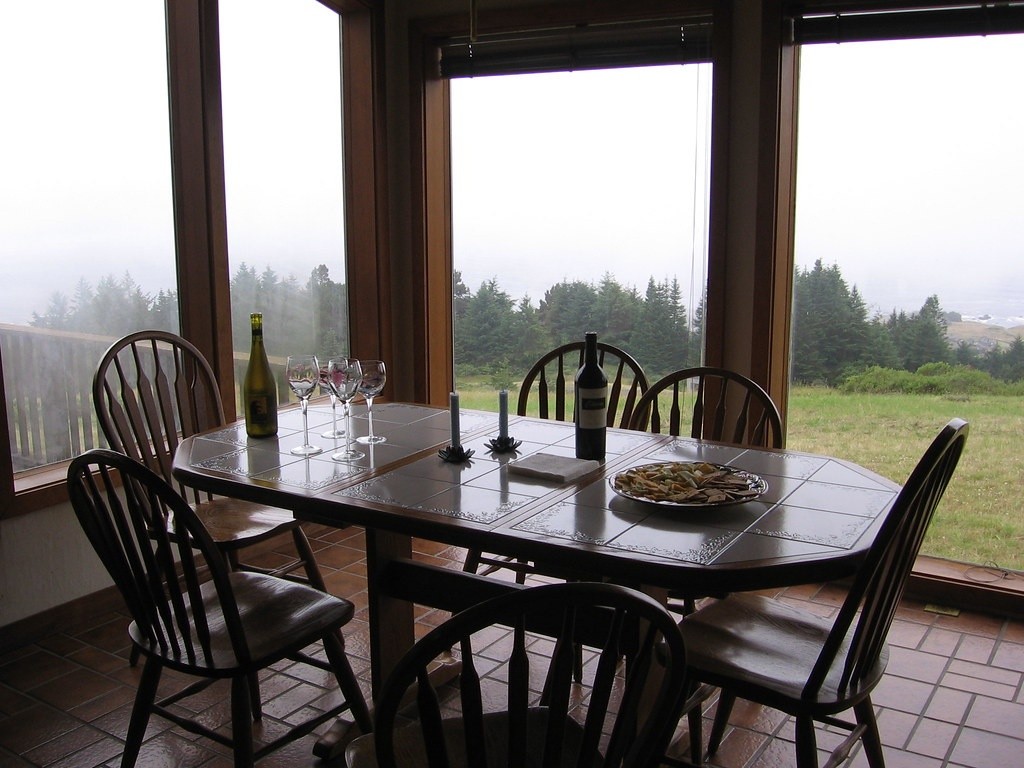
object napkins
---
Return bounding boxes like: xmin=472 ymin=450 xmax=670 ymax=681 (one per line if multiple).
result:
xmin=508 ymin=452 xmax=600 ymax=483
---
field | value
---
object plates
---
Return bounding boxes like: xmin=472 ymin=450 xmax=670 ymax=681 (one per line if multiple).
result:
xmin=609 ymin=461 xmax=770 ymax=515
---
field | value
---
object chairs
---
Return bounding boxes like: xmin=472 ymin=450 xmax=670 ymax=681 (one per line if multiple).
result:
xmin=93 ymin=330 xmax=345 ymax=668
xmin=658 ymin=416 xmax=969 ymax=768
xmin=68 ymin=448 xmax=372 ymax=768
xmin=345 ymin=582 xmax=688 ymax=768
xmin=445 ymin=342 xmax=784 ymax=685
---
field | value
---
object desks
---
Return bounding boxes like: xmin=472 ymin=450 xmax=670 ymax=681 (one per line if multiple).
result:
xmin=173 ymin=402 xmax=902 ymax=768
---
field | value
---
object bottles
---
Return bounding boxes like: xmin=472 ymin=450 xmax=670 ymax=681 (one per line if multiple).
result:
xmin=574 ymin=331 xmax=608 ymax=461
xmin=244 ymin=312 xmax=277 ymax=438
xmin=328 ymin=358 xmax=365 ymax=462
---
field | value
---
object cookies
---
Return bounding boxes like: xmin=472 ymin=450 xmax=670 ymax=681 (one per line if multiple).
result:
xmin=614 ymin=462 xmax=759 ymax=503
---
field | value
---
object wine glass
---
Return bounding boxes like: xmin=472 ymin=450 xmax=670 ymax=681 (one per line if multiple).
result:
xmin=286 ymin=355 xmax=323 ymax=456
xmin=317 ymin=358 xmax=346 ymax=440
xmin=355 ymin=360 xmax=387 ymax=445
